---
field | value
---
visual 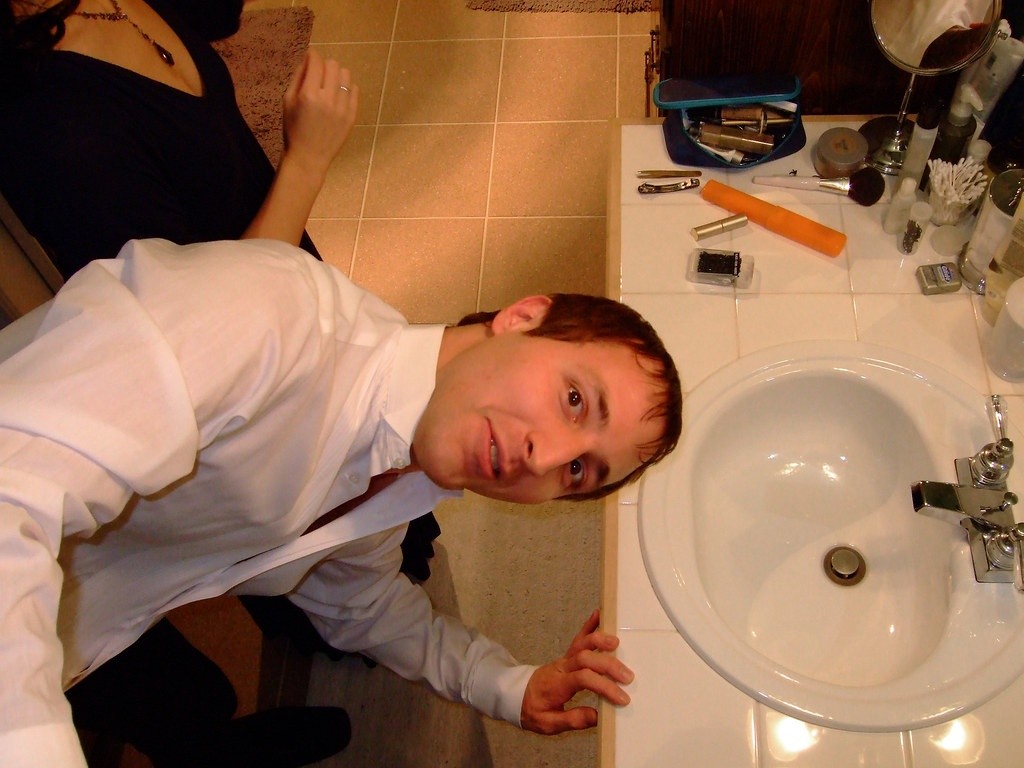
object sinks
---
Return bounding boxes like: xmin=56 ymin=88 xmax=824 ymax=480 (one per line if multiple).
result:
xmin=636 ymin=335 xmax=1019 ymax=736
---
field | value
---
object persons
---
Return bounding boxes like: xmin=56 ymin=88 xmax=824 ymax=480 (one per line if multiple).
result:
xmin=0 ymin=0 xmax=449 ymax=654
xmin=0 ymin=238 xmax=683 ymax=768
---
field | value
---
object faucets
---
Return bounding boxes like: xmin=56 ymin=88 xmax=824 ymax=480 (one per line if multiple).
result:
xmin=911 ymin=481 xmax=1017 ymax=532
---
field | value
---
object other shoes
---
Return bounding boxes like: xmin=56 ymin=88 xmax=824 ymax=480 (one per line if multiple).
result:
xmin=220 ymin=705 xmax=351 ymax=768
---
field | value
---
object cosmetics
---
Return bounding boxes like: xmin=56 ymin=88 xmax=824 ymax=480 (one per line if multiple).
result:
xmin=682 ymin=100 xmax=797 ymax=166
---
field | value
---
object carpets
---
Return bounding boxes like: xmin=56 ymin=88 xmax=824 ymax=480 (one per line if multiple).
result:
xmin=214 ymin=6 xmax=316 ymax=171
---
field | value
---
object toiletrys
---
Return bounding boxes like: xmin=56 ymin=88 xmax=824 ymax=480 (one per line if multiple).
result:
xmin=958 ymin=169 xmax=1024 ymax=382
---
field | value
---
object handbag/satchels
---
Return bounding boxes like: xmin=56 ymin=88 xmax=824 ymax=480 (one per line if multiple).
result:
xmin=652 ymin=70 xmax=804 ymax=170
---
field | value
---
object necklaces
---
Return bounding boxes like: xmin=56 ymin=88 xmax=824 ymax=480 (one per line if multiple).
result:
xmin=73 ymin=0 xmax=176 ymax=66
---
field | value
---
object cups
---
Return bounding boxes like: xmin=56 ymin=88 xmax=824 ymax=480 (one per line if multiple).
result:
xmin=962 ymin=169 xmax=1024 ymax=292
xmin=986 ymin=277 xmax=1024 ymax=383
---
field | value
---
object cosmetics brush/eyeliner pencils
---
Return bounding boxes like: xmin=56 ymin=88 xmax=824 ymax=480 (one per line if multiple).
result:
xmin=752 ymin=167 xmax=887 ymax=207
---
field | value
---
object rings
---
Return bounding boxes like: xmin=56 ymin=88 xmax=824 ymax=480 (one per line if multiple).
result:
xmin=341 ymin=86 xmax=351 ymax=94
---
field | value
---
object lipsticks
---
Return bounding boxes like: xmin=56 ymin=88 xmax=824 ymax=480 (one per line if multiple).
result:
xmin=691 ymin=213 xmax=748 ymax=241
